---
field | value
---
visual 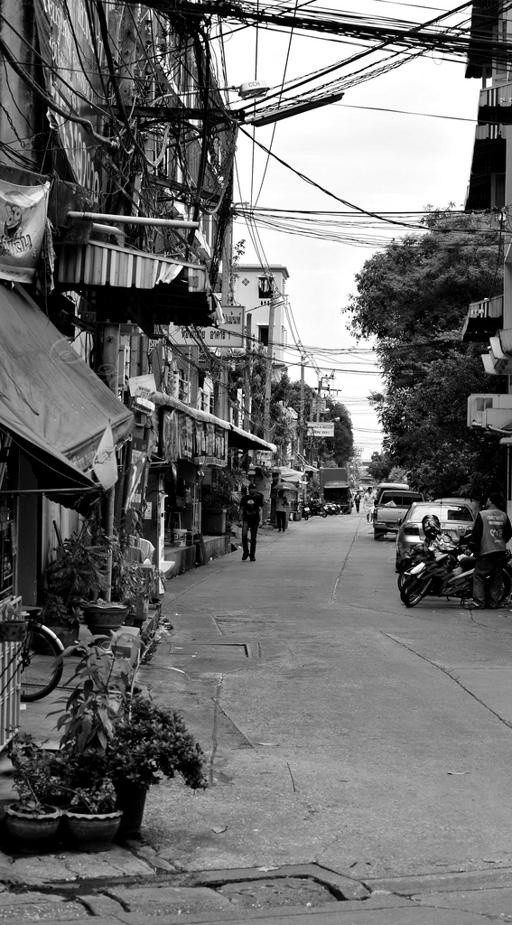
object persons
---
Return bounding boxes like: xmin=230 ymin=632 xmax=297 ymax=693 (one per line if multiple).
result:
xmin=363 ymin=487 xmax=377 ymax=525
xmin=464 ymin=495 xmax=512 ymax=607
xmin=273 ymin=491 xmax=289 ymax=533
xmin=354 ymin=491 xmax=361 ymax=513
xmin=238 ymin=484 xmax=265 ymax=561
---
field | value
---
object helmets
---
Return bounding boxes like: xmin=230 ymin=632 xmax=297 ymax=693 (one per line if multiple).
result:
xmin=421 ymin=514 xmax=441 ymax=540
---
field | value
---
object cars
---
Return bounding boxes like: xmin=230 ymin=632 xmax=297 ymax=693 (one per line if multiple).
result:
xmin=373 ymin=481 xmax=478 ymax=568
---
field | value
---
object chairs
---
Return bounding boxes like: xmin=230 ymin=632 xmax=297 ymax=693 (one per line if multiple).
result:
xmin=78 ymin=534 xmax=176 ymax=596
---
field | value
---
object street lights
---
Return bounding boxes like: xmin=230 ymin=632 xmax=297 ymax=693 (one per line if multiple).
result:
xmin=297 ymin=408 xmax=330 ymax=462
xmin=326 ymin=416 xmax=342 ymax=423
xmin=261 ymin=357 xmax=310 ymax=443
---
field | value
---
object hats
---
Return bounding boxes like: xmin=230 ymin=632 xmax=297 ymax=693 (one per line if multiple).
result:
xmin=248 ymin=483 xmax=257 ymax=489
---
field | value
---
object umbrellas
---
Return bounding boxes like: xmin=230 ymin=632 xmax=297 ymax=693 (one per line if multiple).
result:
xmin=273 ymin=482 xmax=298 ymax=506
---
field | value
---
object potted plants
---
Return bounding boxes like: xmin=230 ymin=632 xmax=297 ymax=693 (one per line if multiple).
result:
xmin=224 ymin=466 xmax=248 ymax=503
xmin=35 ymin=524 xmax=164 ymax=657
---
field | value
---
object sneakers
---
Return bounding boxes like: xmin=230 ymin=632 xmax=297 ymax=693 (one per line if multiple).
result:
xmin=241 ymin=553 xmax=257 ymax=561
xmin=460 ymin=597 xmax=498 ymax=610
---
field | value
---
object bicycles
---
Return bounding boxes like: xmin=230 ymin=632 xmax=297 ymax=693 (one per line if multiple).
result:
xmin=19 ymin=604 xmax=64 ymax=704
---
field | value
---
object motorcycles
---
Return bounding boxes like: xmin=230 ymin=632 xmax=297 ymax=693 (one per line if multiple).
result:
xmin=392 ymin=515 xmax=512 ymax=608
xmin=300 ymin=497 xmax=340 ymax=520
